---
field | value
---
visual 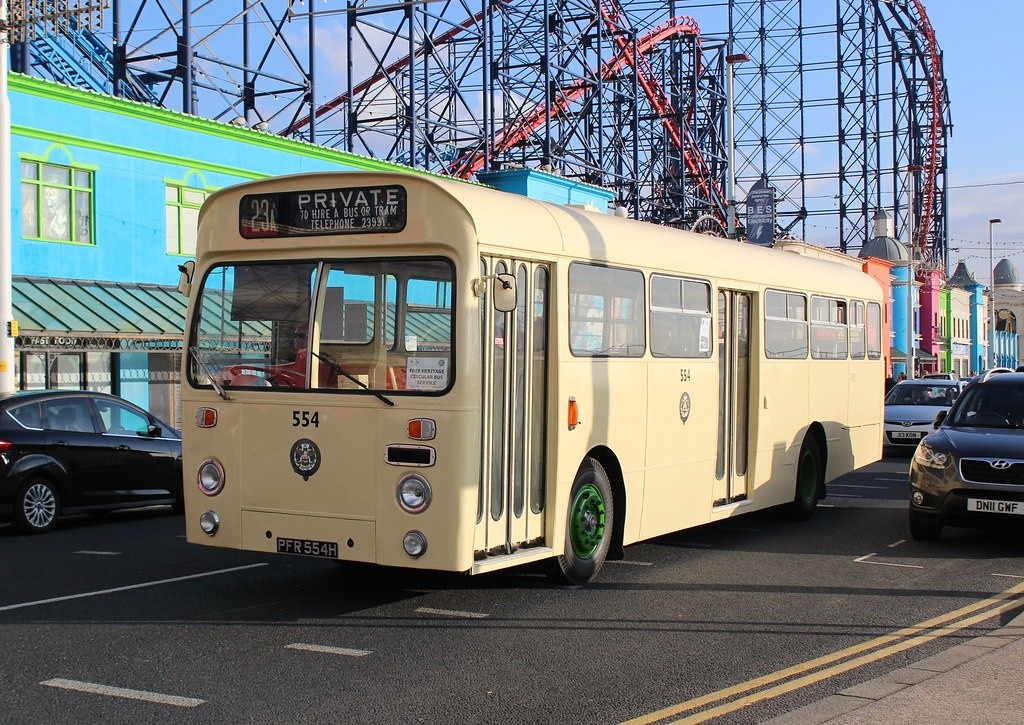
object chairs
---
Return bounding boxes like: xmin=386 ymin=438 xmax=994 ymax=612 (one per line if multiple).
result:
xmin=56 ymin=407 xmax=83 ymax=431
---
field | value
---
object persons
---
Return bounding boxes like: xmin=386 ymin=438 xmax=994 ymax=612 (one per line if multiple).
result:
xmin=886 ymin=367 xmax=995 ymax=394
xmin=263 ymin=323 xmax=339 ymax=389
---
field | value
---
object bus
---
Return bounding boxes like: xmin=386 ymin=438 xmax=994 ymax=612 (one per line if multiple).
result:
xmin=180 ymin=168 xmax=885 ymax=587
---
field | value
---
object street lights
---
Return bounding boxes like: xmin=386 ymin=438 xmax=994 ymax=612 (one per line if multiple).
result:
xmin=725 ymin=54 xmax=751 ymax=239
xmin=988 ymin=217 xmax=1002 ymax=372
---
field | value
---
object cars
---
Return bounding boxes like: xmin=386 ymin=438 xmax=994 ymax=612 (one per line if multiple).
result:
xmin=883 ymin=379 xmax=971 ymax=457
xmin=0 ymin=391 xmax=185 ymax=533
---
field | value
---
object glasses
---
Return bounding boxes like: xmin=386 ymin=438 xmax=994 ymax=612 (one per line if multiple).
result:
xmin=293 ymin=332 xmax=308 ymax=340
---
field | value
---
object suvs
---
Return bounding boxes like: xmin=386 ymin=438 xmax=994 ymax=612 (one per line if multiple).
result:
xmin=908 ymin=368 xmax=1023 ymax=544
